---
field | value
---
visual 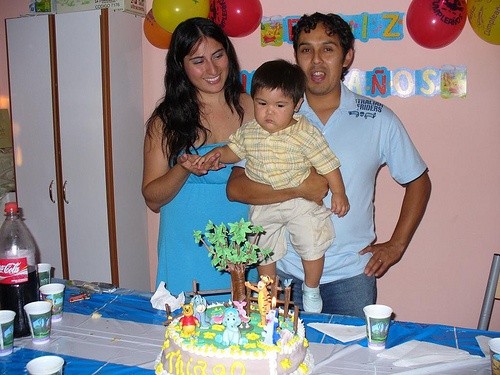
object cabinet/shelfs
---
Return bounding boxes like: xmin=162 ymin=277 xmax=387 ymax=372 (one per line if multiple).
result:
xmin=2 ymin=10 xmax=149 ymax=294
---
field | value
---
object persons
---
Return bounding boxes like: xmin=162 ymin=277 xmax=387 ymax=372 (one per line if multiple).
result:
xmin=225 ymin=14 xmax=432 ymax=319
xmin=143 ymin=15 xmax=259 ymax=304
xmin=191 ymin=58 xmax=351 ymax=317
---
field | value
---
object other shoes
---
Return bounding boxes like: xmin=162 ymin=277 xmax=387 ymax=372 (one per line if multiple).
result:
xmin=301 ymin=281 xmax=323 ymax=313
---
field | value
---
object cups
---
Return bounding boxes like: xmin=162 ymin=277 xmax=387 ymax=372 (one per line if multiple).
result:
xmin=488 ymin=337 xmax=500 ymax=375
xmin=24 ymin=301 xmax=52 ymax=345
xmin=39 ymin=283 xmax=65 ymax=323
xmin=36 ymin=263 xmax=51 ymax=287
xmin=363 ymin=304 xmax=392 ymax=351
xmin=0 ymin=309 xmax=17 ymax=357
xmin=25 ymin=356 xmax=64 ymax=375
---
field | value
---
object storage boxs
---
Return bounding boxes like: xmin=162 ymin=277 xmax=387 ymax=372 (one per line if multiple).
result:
xmin=0 ymin=109 xmax=15 ymax=193
xmin=34 ymin=0 xmax=147 ymax=16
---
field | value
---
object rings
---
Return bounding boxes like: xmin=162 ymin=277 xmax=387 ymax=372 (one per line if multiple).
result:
xmin=378 ymin=259 xmax=382 ymax=263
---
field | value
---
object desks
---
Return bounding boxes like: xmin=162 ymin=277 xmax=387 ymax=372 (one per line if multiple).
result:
xmin=0 ymin=280 xmax=500 ymax=375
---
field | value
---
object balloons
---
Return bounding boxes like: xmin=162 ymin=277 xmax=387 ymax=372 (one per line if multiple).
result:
xmin=466 ymin=0 xmax=500 ymax=46
xmin=209 ymin=0 xmax=264 ymax=38
xmin=407 ymin=0 xmax=469 ymax=48
xmin=144 ymin=7 xmax=180 ymax=49
xmin=152 ymin=0 xmax=210 ymax=32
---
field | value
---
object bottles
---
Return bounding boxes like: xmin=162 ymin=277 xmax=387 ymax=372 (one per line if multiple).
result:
xmin=0 ymin=201 xmax=38 ymax=339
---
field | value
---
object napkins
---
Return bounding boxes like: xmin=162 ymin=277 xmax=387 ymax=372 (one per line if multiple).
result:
xmin=475 ymin=335 xmax=493 ymax=357
xmin=376 ymin=339 xmax=470 ymax=367
xmin=150 ymin=281 xmax=185 ymax=312
xmin=306 ymin=322 xmax=368 ymax=343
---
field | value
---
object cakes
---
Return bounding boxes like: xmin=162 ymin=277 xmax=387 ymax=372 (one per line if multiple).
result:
xmin=154 ymin=299 xmax=313 ymax=375
